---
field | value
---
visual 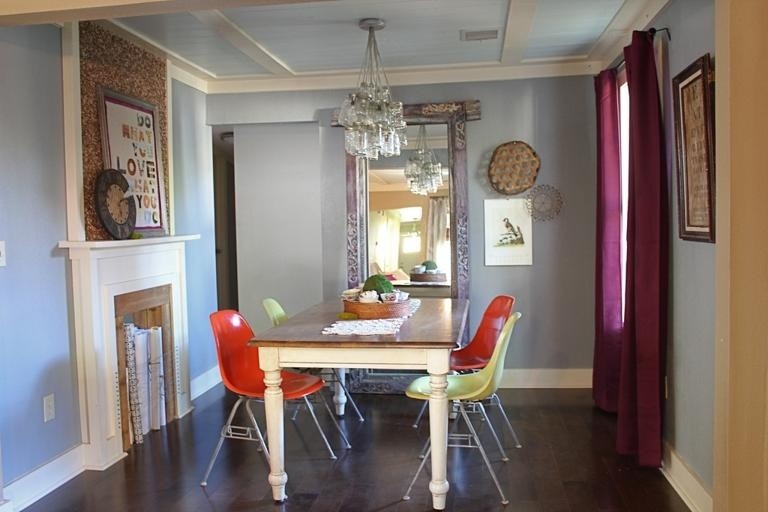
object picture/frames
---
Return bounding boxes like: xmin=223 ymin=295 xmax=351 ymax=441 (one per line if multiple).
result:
xmin=97 ymin=83 xmax=171 ymax=238
xmin=672 ymin=53 xmax=716 ymax=243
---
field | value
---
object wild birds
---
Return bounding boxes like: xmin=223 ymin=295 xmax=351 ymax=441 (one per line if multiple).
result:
xmin=501 ymin=217 xmax=518 ymax=236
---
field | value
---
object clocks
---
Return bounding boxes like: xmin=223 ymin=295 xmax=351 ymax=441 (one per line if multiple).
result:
xmin=96 ymin=169 xmax=136 ymax=240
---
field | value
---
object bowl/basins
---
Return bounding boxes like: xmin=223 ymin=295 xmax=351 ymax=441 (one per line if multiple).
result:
xmin=342 ymin=288 xmax=410 ymax=304
xmin=415 ymin=265 xmax=437 ymax=274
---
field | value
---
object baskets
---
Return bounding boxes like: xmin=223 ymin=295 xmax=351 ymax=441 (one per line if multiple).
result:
xmin=342 ymin=297 xmax=412 ymax=319
xmin=410 ymin=272 xmax=447 ymax=282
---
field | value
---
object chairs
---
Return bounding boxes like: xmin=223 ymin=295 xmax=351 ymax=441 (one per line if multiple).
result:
xmin=202 ymin=297 xmax=364 ymax=500
xmin=404 ymin=294 xmax=522 ymax=509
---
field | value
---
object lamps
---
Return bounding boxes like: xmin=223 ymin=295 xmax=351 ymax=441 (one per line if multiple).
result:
xmin=336 ymin=17 xmax=409 ymax=159
xmin=404 ymin=124 xmax=443 ymax=195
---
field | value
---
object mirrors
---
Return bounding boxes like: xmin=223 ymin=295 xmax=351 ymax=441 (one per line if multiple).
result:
xmin=332 ymin=99 xmax=480 ymax=393
